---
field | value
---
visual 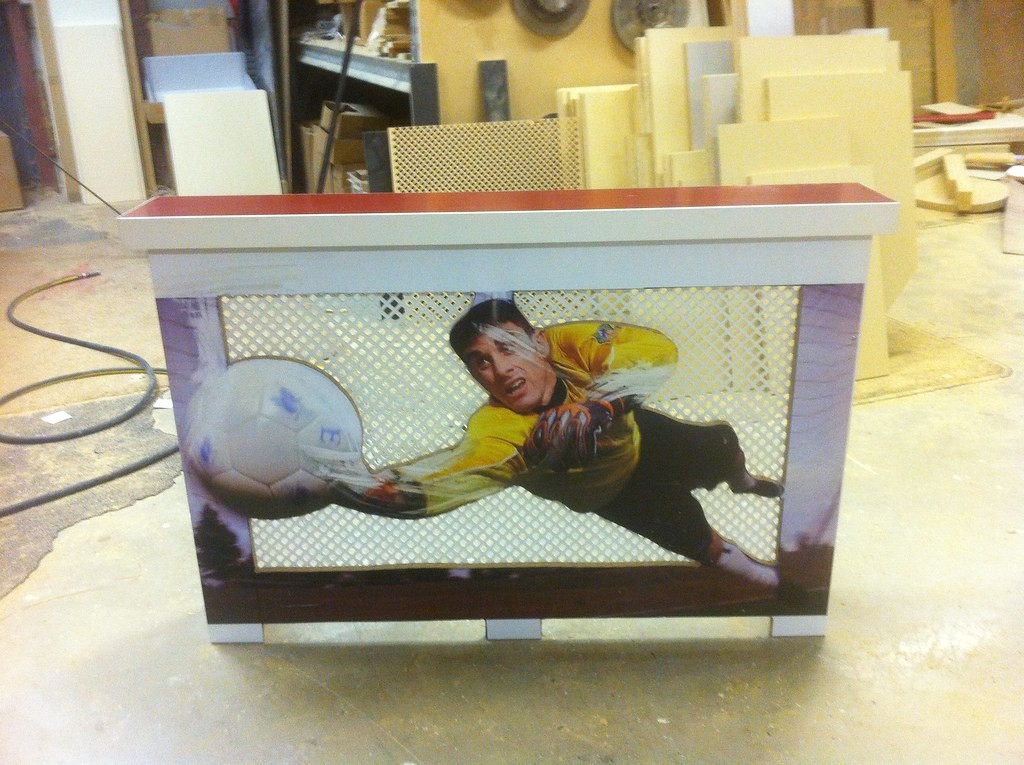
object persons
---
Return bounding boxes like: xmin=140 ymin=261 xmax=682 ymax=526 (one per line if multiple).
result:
xmin=329 ymin=298 xmax=784 ymax=590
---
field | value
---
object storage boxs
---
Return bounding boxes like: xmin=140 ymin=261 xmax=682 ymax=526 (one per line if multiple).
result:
xmin=161 ymin=89 xmax=284 ymax=196
xmin=793 ymin=1 xmax=961 ymax=116
xmin=136 ymin=8 xmax=230 ymax=57
xmin=1 ymin=132 xmax=23 ymax=211
xmin=297 ymin=100 xmax=389 ymax=195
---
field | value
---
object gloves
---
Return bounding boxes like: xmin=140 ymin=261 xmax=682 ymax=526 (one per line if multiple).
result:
xmin=525 ymin=396 xmax=626 ymax=471
xmin=332 ymin=471 xmax=431 ymax=521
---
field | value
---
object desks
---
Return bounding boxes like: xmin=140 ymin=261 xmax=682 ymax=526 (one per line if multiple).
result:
xmin=912 ymin=114 xmax=1022 ymax=173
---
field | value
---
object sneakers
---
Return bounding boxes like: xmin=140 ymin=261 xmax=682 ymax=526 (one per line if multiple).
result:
xmin=733 ymin=476 xmax=787 ymax=503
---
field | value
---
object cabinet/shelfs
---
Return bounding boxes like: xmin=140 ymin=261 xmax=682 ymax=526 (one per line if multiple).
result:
xmin=286 ymin=0 xmax=750 ymax=195
xmin=118 ymin=1 xmax=294 ymax=197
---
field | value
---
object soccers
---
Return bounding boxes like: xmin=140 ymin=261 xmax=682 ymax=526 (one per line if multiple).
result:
xmin=183 ymin=356 xmax=364 ymax=521
